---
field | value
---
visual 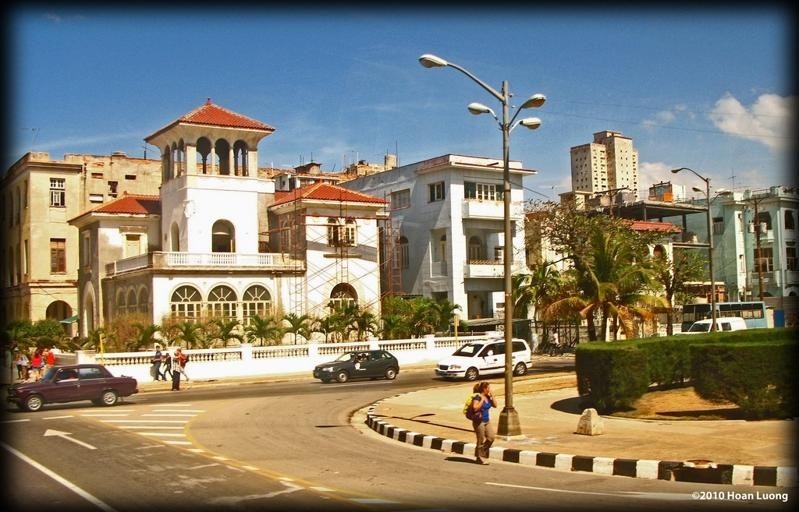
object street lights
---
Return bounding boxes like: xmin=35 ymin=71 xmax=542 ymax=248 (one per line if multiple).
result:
xmin=418 ymin=53 xmax=571 ymax=443
xmin=670 ymin=167 xmax=734 ymax=333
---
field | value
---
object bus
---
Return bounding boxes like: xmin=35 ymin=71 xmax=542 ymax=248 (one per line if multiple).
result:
xmin=681 ymin=300 xmax=770 ymax=333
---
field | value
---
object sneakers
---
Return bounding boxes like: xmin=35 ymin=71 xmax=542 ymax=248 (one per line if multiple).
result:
xmin=476 ymin=456 xmax=489 ymax=465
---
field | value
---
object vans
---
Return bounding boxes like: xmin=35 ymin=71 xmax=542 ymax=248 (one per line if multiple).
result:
xmin=687 ymin=316 xmax=748 ymax=335
xmin=433 ymin=337 xmax=533 ymax=382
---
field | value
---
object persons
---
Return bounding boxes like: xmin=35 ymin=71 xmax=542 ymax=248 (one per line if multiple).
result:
xmin=472 ymin=381 xmax=498 ymax=465
xmin=68 ymin=370 xmax=77 ymax=379
xmin=15 ymin=348 xmax=56 ymax=382
xmin=548 ymin=329 xmax=564 ymax=357
xmin=172 ymin=352 xmax=182 ymax=391
xmin=162 ymin=352 xmax=173 ymax=381
xmin=176 ymin=347 xmax=189 ymax=381
xmin=361 ymin=354 xmax=367 ymax=360
xmin=151 ymin=345 xmax=167 ymax=382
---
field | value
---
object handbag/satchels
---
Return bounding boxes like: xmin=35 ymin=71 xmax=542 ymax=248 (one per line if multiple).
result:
xmin=463 ymin=392 xmax=482 ymax=423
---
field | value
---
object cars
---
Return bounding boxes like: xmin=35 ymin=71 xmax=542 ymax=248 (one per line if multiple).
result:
xmin=313 ymin=348 xmax=400 ymax=384
xmin=5 ymin=362 xmax=139 ymax=413
